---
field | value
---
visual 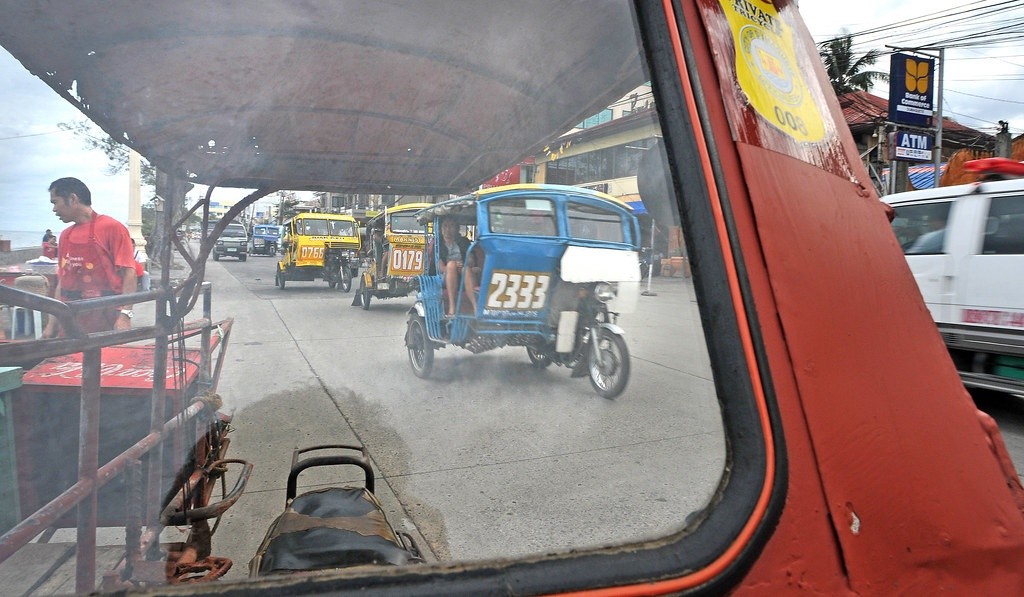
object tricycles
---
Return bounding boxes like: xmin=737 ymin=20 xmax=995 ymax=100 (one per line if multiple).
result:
xmin=0 ymin=0 xmax=1024 ymax=597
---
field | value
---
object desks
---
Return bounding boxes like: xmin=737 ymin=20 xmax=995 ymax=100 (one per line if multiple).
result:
xmin=0 ymin=258 xmax=60 ymax=339
xmin=24 ymin=346 xmax=213 ymax=530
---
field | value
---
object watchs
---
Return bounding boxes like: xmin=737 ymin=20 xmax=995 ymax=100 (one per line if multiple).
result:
xmin=121 ymin=309 xmax=135 ymax=319
xmin=380 ymin=227 xmax=389 ymax=276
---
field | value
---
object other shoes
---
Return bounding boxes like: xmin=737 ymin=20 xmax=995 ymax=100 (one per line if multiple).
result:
xmin=447 ymin=311 xmax=454 ymax=318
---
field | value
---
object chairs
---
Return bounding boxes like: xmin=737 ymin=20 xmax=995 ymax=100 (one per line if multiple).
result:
xmin=9 ymin=276 xmax=51 ymax=341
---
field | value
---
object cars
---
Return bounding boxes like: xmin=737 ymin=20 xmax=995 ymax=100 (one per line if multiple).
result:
xmin=878 ymin=179 xmax=1024 ymax=401
xmin=176 ymin=219 xmax=219 ymax=240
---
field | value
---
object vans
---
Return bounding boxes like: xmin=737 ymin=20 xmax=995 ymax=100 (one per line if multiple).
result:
xmin=212 ymin=224 xmax=248 ymax=261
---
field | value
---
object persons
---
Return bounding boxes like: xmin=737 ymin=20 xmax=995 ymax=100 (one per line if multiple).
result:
xmin=41 ymin=176 xmax=150 ymax=338
xmin=904 ymin=206 xmax=949 ymax=252
xmin=428 ymin=214 xmax=477 ymax=317
xmin=282 ymin=223 xmax=299 ymax=258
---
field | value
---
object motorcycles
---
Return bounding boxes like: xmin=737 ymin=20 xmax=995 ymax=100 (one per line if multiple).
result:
xmin=275 ymin=213 xmax=362 ymax=293
xmin=404 ymin=182 xmax=643 ymax=399
xmin=358 ymin=202 xmax=472 ymax=309
xmin=248 ymin=225 xmax=283 ymax=257
